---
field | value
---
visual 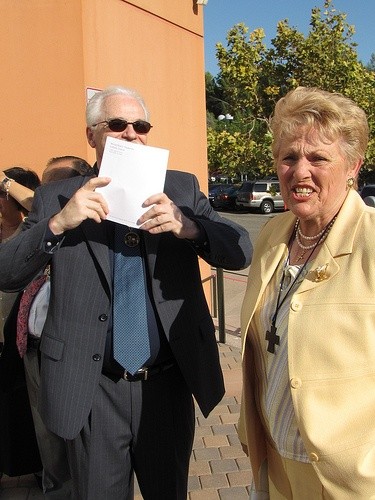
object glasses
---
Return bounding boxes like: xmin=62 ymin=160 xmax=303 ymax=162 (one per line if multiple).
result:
xmin=91 ymin=118 xmax=154 ymax=135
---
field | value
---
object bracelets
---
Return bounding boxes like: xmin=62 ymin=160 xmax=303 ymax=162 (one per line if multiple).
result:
xmin=1 ymin=176 xmax=14 ymax=200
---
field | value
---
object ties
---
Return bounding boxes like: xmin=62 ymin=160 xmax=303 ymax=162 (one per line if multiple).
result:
xmin=112 ymin=223 xmax=151 ymax=376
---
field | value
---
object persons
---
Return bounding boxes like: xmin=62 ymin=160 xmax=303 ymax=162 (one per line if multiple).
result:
xmin=0 ymin=87 xmax=252 ymax=500
xmin=233 ymin=86 xmax=375 ymax=500
xmin=0 ymin=155 xmax=90 ymax=478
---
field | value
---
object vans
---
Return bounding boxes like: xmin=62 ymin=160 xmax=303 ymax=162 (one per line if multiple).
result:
xmin=235 ymin=179 xmax=287 ymax=214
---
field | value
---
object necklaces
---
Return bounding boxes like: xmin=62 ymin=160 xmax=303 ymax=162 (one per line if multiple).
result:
xmin=266 ymin=210 xmax=337 ymax=355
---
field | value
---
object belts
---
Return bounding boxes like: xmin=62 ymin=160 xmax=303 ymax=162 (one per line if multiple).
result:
xmin=101 ymin=360 xmax=153 ymax=381
xmin=26 ymin=335 xmax=41 ymax=352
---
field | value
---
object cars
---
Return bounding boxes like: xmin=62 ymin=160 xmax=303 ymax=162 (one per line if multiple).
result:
xmin=208 ymin=183 xmax=241 ymax=211
xmin=359 ymin=185 xmax=375 ymax=199
